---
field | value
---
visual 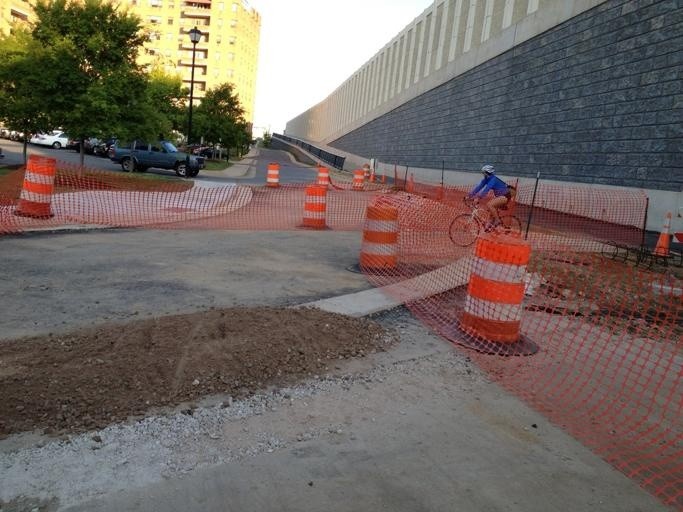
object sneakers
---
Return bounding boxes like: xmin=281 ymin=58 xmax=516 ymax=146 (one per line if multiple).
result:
xmin=483 ymin=221 xmax=502 ymax=231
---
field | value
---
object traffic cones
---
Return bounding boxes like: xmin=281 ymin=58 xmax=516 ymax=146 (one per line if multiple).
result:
xmin=656 ymin=212 xmax=672 ymax=254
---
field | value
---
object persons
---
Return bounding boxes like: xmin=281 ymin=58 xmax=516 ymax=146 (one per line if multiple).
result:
xmin=465 ymin=164 xmax=512 ymax=232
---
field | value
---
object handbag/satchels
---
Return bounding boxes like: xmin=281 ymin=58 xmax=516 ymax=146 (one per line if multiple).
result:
xmin=510 ymin=186 xmax=516 ymax=196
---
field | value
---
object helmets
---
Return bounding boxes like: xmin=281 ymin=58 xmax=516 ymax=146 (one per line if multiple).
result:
xmin=481 ymin=165 xmax=495 ymax=174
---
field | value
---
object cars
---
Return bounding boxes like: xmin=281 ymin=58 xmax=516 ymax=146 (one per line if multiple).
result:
xmin=29 ymin=129 xmax=79 ymax=150
xmin=109 ymin=139 xmax=206 ymax=177
xmin=73 ymin=137 xmax=114 ymax=157
xmin=185 ymin=143 xmax=227 ymax=159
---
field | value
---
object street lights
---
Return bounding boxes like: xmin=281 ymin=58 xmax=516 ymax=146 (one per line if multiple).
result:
xmin=186 ymin=27 xmax=208 ymax=155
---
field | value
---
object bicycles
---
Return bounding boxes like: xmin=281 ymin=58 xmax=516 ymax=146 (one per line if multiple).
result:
xmin=448 ymin=196 xmax=523 ymax=245
